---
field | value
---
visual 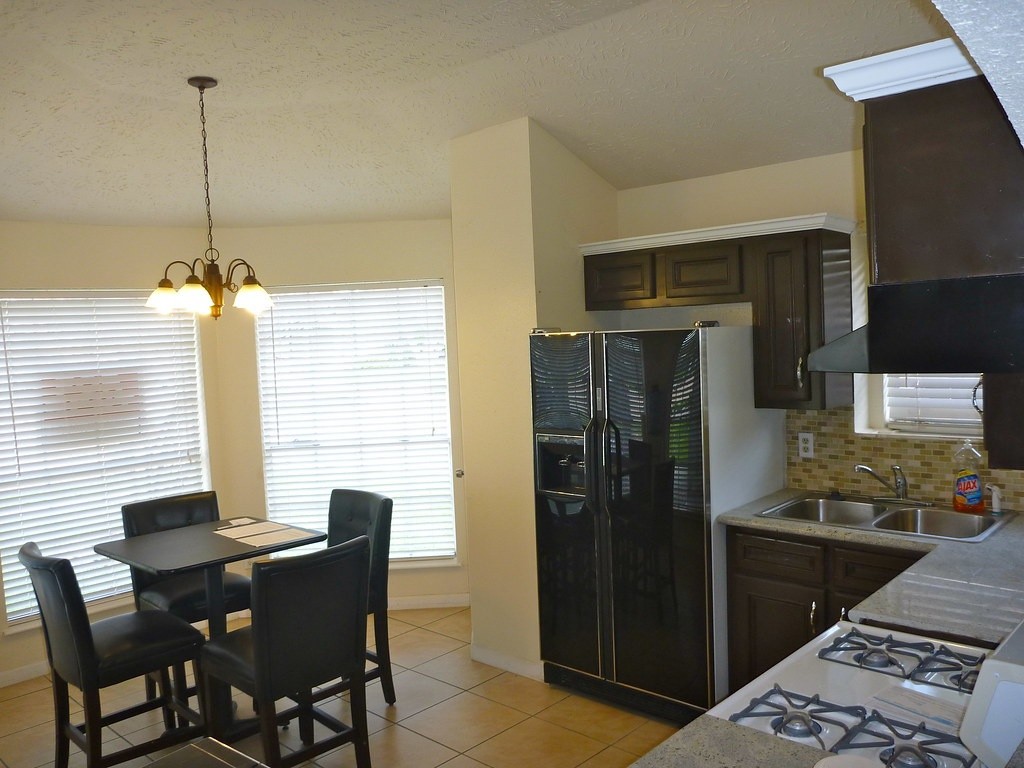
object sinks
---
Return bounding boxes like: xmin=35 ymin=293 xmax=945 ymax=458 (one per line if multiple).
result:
xmin=874 ymin=505 xmax=1004 ymax=543
xmin=760 ymin=495 xmax=888 ymax=531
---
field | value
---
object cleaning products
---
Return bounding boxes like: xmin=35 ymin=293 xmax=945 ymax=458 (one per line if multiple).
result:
xmin=952 ymin=440 xmax=983 ymax=512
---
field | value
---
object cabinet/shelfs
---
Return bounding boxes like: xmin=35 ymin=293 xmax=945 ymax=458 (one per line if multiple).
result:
xmin=749 ymin=228 xmax=853 ymax=411
xmin=584 ymin=236 xmax=750 ymax=312
xmin=726 ymin=524 xmax=927 ymax=697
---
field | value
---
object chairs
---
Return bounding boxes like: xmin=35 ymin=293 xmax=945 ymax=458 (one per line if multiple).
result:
xmin=253 ymin=489 xmax=397 ymax=730
xmin=120 ymin=490 xmax=254 ymax=728
xmin=201 ymin=535 xmax=372 ymax=768
xmin=17 ymin=541 xmax=206 ymax=768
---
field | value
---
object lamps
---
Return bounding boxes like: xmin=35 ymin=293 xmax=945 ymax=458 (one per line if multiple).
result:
xmin=145 ymin=76 xmax=274 ymax=321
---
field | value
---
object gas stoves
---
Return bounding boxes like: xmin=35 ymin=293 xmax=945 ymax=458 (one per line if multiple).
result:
xmin=706 ymin=621 xmax=1023 ymax=767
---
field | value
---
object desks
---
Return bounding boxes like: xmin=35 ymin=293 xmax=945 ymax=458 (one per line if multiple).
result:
xmin=93 ymin=517 xmax=327 ymax=744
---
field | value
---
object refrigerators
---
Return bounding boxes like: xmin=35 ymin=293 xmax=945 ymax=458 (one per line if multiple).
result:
xmin=529 ymin=323 xmax=788 ymax=726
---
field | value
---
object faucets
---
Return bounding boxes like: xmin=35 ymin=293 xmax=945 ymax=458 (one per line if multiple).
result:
xmin=851 ymin=462 xmax=908 ymax=499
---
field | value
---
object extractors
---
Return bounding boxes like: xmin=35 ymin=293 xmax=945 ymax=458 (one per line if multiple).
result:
xmin=807 ymin=275 xmax=1024 ymax=373
